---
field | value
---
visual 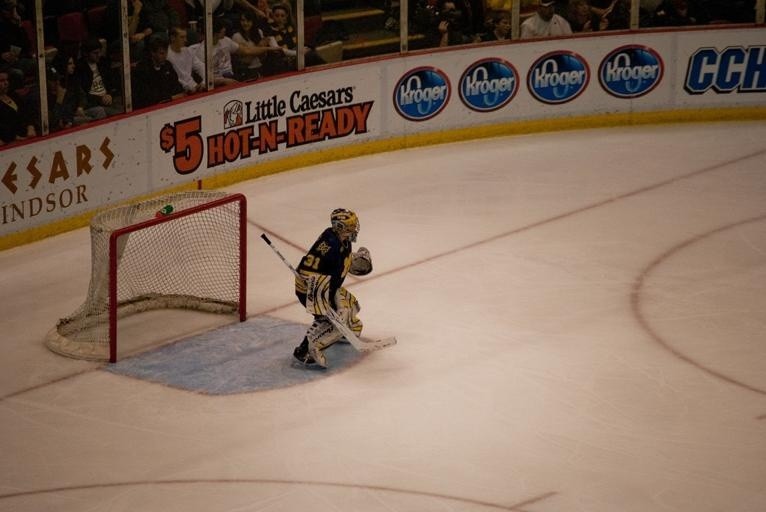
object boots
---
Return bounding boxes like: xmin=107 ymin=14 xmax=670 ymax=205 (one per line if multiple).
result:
xmin=293 ymin=335 xmax=328 ymax=366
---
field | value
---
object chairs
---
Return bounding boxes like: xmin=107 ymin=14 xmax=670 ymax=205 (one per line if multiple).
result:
xmin=18 ymin=5 xmax=142 ymax=82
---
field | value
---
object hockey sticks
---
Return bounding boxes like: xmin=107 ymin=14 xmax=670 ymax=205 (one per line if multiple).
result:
xmin=260 ymin=233 xmax=396 ymax=353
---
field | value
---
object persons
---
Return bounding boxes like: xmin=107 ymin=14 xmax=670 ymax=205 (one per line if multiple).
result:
xmin=293 ymin=208 xmax=363 ymax=364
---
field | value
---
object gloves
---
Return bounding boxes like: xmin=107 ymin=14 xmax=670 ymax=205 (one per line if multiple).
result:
xmin=348 ymin=247 xmax=372 ymax=276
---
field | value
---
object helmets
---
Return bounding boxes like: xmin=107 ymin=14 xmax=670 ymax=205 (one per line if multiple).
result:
xmin=330 ymin=208 xmax=360 ymax=243
